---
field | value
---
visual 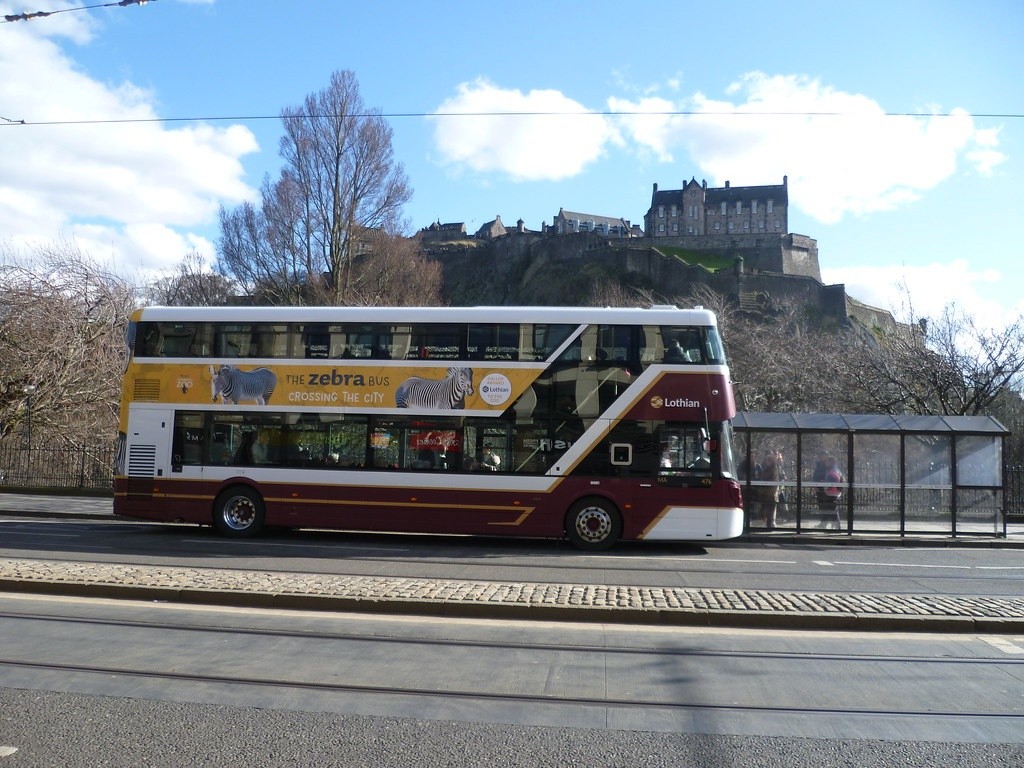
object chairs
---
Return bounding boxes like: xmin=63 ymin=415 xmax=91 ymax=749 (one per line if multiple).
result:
xmin=294 ymin=451 xmax=401 ymax=470
xmin=222 ymin=340 xmax=488 ymax=361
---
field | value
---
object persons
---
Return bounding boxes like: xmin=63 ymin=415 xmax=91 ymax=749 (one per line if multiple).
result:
xmin=177 ymin=340 xmax=849 ymax=531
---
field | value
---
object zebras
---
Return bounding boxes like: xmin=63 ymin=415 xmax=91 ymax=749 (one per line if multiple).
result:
xmin=394 ymin=367 xmax=475 ymax=410
xmin=209 ymin=364 xmax=276 ymax=407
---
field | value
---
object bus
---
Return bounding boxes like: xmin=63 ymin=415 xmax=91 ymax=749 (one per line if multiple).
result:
xmin=114 ymin=306 xmax=744 ymax=552
